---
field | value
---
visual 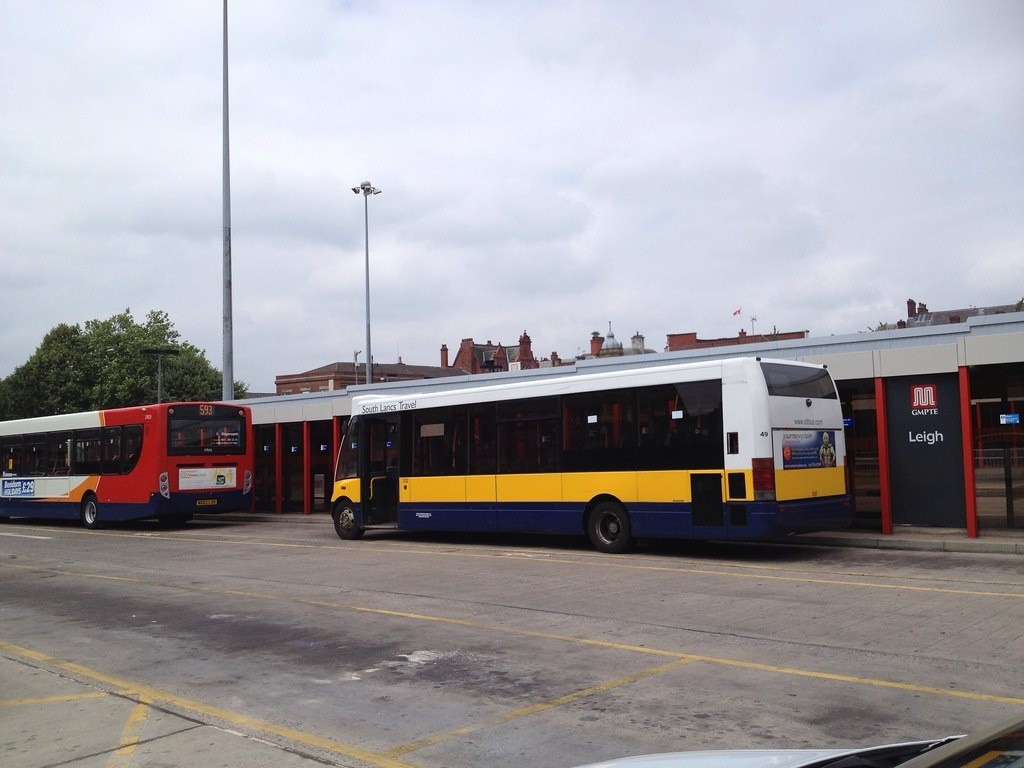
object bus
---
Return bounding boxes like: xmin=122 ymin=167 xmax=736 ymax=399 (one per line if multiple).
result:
xmin=337 ymin=354 xmax=850 ymax=554
xmin=1 ymin=401 xmax=255 ymax=529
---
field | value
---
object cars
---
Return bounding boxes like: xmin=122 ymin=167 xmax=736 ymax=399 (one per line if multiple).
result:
xmin=574 ymin=714 xmax=1024 ymax=768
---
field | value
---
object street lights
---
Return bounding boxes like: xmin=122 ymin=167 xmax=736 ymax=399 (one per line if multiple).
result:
xmin=138 ymin=348 xmax=181 ymax=405
xmin=351 ymin=180 xmax=383 ymax=380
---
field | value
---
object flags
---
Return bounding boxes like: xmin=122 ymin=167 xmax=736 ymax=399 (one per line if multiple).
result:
xmin=733 ymin=310 xmax=740 ymax=315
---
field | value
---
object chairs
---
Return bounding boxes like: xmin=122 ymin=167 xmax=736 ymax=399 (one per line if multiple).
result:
xmin=44 ymin=454 xmax=136 ymax=476
